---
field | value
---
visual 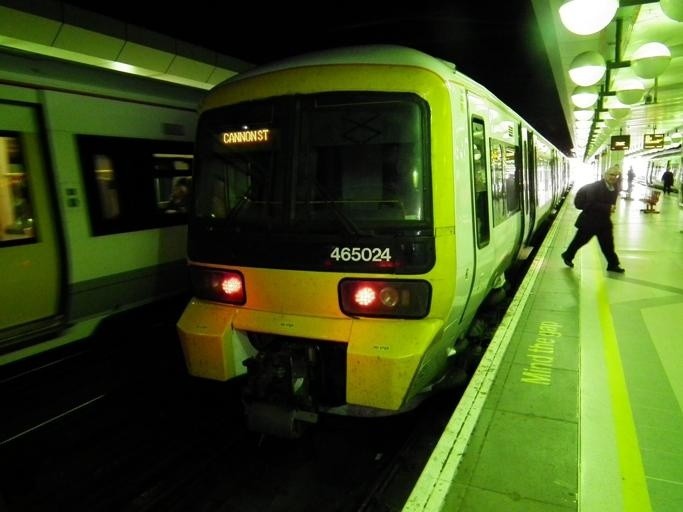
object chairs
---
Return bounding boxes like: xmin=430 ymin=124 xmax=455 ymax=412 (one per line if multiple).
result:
xmin=638 ymin=190 xmax=661 ymax=213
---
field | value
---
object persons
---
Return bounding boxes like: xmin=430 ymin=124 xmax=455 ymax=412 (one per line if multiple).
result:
xmin=662 ymin=168 xmax=673 ymax=194
xmin=561 ymin=167 xmax=625 ymax=272
xmin=627 ymin=166 xmax=634 ymax=188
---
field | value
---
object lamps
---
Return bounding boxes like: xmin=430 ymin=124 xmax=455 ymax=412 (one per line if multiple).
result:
xmin=550 ymin=0 xmax=683 ymax=164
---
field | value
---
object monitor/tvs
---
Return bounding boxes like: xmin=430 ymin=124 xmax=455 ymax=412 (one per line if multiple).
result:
xmin=643 ymin=134 xmax=664 ymax=149
xmin=610 ymin=135 xmax=630 ymax=150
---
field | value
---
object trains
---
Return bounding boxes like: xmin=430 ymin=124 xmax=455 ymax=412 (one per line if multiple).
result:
xmin=168 ymin=43 xmax=574 ymax=443
xmin=0 ymin=79 xmax=249 ymax=383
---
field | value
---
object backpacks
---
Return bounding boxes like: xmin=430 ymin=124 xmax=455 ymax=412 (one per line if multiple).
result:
xmin=574 ymin=180 xmax=600 ymax=210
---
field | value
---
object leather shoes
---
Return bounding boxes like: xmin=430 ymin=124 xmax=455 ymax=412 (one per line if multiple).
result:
xmin=561 ymin=250 xmax=575 ymax=268
xmin=605 ymin=263 xmax=626 ymax=274
